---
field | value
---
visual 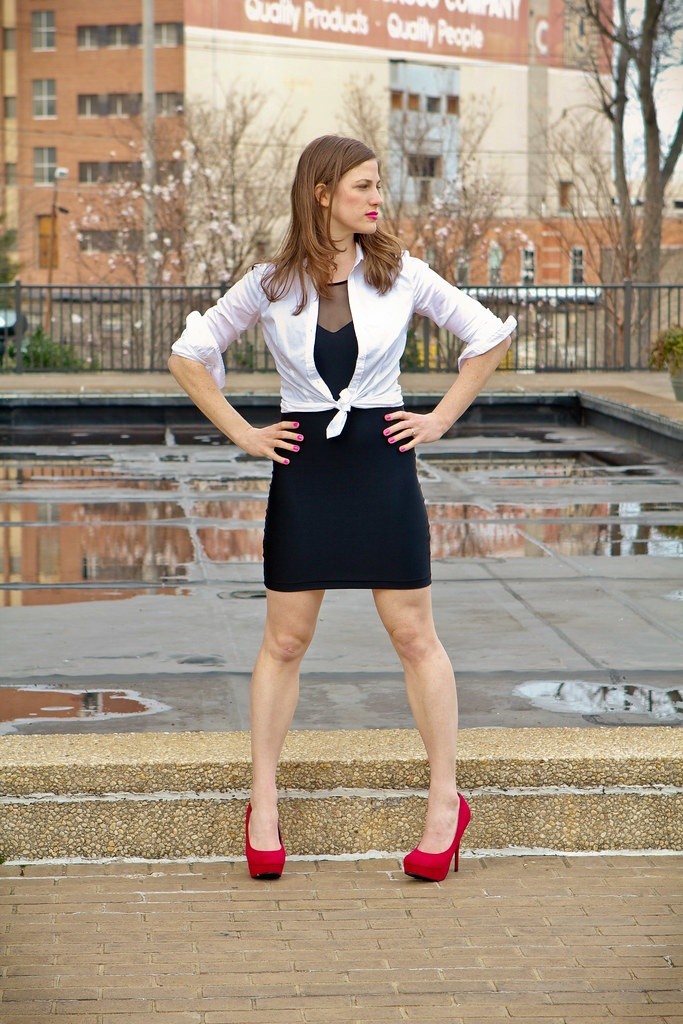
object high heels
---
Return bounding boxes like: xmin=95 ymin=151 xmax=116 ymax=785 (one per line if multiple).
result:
xmin=245 ymin=803 xmax=287 ymax=880
xmin=403 ymin=792 xmax=472 ymax=882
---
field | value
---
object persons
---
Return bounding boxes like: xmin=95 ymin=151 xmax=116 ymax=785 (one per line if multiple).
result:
xmin=168 ymin=136 xmax=516 ymax=882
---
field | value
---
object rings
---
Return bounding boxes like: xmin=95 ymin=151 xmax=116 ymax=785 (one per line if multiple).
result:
xmin=411 ymin=428 xmax=416 ymax=436
